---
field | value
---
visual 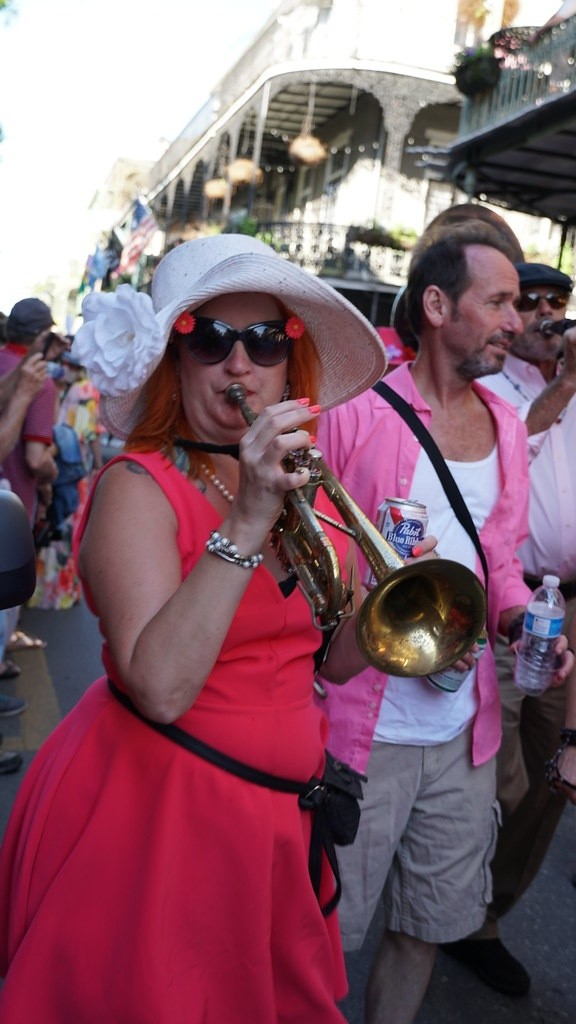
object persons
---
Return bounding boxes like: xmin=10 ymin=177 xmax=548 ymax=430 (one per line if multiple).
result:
xmin=315 ymin=205 xmax=576 ymax=1024
xmin=0 ymin=234 xmax=437 ymax=1024
xmin=0 ymin=298 xmax=115 ymax=719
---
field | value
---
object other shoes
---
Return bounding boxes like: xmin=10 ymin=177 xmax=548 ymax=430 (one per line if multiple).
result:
xmin=547 ymin=759 xmax=576 ymax=805
xmin=0 ymin=750 xmax=22 ymax=773
xmin=0 ymin=661 xmax=23 ymax=678
xmin=0 ymin=694 xmax=28 ymax=717
xmin=437 ymin=936 xmax=530 ymax=1000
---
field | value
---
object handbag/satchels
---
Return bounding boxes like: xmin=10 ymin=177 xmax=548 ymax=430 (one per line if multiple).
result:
xmin=322 ymin=748 xmax=368 ymax=846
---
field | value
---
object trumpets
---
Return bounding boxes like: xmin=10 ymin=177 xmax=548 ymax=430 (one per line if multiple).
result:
xmin=222 ymin=380 xmax=489 ymax=679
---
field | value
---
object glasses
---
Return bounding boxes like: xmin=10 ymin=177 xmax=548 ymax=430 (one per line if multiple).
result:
xmin=173 ymin=312 xmax=305 ymax=367
xmin=517 ymin=291 xmax=570 ymax=311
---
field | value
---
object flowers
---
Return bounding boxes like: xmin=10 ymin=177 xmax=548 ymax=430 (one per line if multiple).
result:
xmin=171 ymin=311 xmax=196 ymax=335
xmin=454 ymin=41 xmax=495 ymax=72
xmin=284 ymin=316 xmax=305 ymax=341
xmin=69 ymin=284 xmax=168 ymax=399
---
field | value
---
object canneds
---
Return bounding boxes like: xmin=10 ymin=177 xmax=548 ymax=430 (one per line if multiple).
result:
xmin=361 ymin=497 xmax=430 ymax=591
xmin=426 ymin=631 xmax=487 ymax=693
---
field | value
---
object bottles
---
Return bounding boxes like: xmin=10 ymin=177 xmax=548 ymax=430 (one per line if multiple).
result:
xmin=540 ymin=318 xmax=576 ymax=337
xmin=513 ymin=575 xmax=566 ymax=697
xmin=45 ymin=360 xmax=76 ymax=385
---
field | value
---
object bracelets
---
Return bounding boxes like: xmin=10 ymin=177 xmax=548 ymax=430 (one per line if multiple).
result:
xmin=205 ymin=531 xmax=264 ymax=568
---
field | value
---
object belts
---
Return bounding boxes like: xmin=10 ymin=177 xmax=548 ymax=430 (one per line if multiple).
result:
xmin=523 ymin=577 xmax=576 ymax=602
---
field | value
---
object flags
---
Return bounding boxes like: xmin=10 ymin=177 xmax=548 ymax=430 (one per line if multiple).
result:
xmin=79 ymin=196 xmax=158 ymax=292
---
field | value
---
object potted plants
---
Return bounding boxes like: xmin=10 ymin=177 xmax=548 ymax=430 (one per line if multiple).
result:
xmin=455 ymin=56 xmax=499 ymax=96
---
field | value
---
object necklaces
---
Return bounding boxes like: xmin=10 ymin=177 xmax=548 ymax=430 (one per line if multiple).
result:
xmin=503 ymin=372 xmax=568 ymax=424
xmin=201 ymin=464 xmax=234 ymax=502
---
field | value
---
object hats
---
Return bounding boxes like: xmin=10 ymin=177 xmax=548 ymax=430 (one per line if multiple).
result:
xmin=71 ymin=234 xmax=388 ymax=442
xmin=9 ymin=298 xmax=57 ymax=335
xmin=514 ymin=262 xmax=573 ymax=295
